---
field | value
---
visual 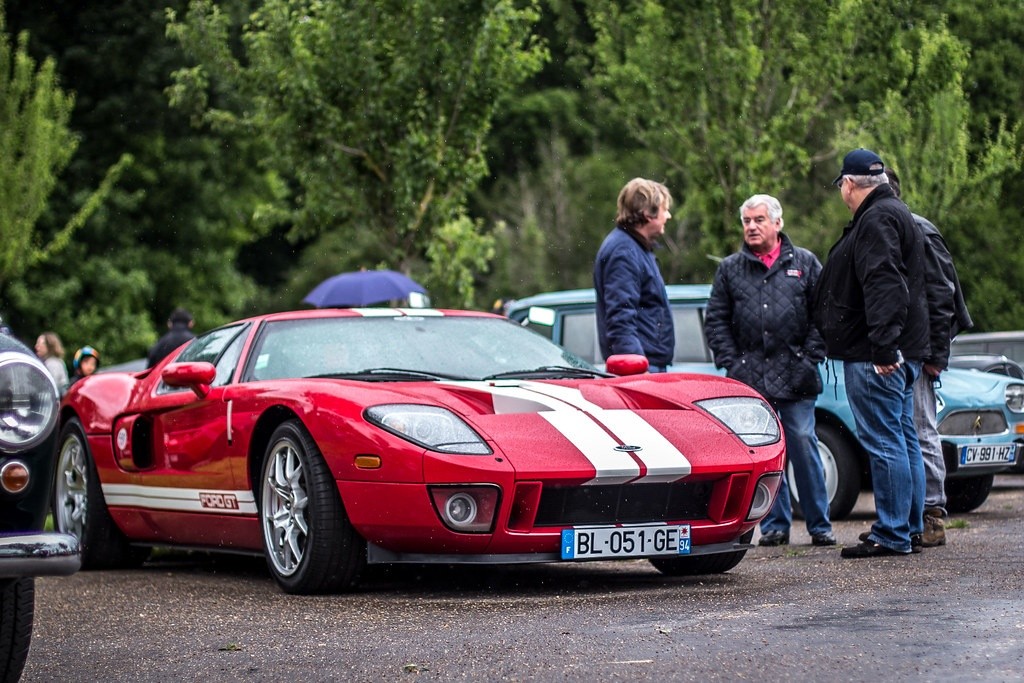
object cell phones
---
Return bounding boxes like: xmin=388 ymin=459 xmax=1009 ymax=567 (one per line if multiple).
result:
xmin=872 ymin=349 xmax=905 ymax=373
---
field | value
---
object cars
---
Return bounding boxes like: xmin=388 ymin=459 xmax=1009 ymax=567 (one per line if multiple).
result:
xmin=947 ymin=330 xmax=1023 ymax=379
xmin=0 ymin=310 xmax=83 ymax=683
xmin=504 ymin=280 xmax=1024 ymax=524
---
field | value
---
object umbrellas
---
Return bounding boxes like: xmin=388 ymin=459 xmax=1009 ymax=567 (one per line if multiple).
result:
xmin=300 ymin=267 xmax=425 ymax=308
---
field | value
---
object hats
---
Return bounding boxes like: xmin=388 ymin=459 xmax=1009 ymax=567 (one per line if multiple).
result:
xmin=170 ymin=306 xmax=193 ymax=322
xmin=832 ymin=148 xmax=884 ymax=186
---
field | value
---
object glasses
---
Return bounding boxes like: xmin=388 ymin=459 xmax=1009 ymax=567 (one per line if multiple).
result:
xmin=837 ymin=178 xmax=853 ymax=188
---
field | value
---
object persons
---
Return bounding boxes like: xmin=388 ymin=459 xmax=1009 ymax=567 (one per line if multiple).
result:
xmin=34 ymin=333 xmax=69 ymax=394
xmin=147 ymin=309 xmax=196 ymax=368
xmin=703 ymin=195 xmax=839 ymax=546
xmin=816 ymin=149 xmax=975 ymax=558
xmin=384 ymin=296 xmax=410 ymax=308
xmin=593 ymin=176 xmax=677 ymax=374
xmin=70 ymin=344 xmax=100 ymax=384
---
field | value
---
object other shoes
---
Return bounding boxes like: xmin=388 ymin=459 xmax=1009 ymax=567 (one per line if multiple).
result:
xmin=840 ymin=540 xmax=897 ymax=558
xmin=858 ymin=532 xmax=923 ymax=552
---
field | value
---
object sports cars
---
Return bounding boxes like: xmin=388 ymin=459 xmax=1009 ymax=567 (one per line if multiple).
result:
xmin=48 ymin=305 xmax=786 ymax=594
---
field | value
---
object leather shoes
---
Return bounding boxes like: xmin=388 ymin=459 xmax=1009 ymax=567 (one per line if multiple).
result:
xmin=812 ymin=531 xmax=837 ymax=545
xmin=758 ymin=530 xmax=789 ymax=546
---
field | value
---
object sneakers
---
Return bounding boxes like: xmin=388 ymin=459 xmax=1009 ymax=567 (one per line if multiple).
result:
xmin=921 ymin=508 xmax=946 ymax=546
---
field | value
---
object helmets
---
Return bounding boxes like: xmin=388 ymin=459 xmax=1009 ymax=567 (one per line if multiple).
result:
xmin=72 ymin=346 xmax=100 ymax=376
